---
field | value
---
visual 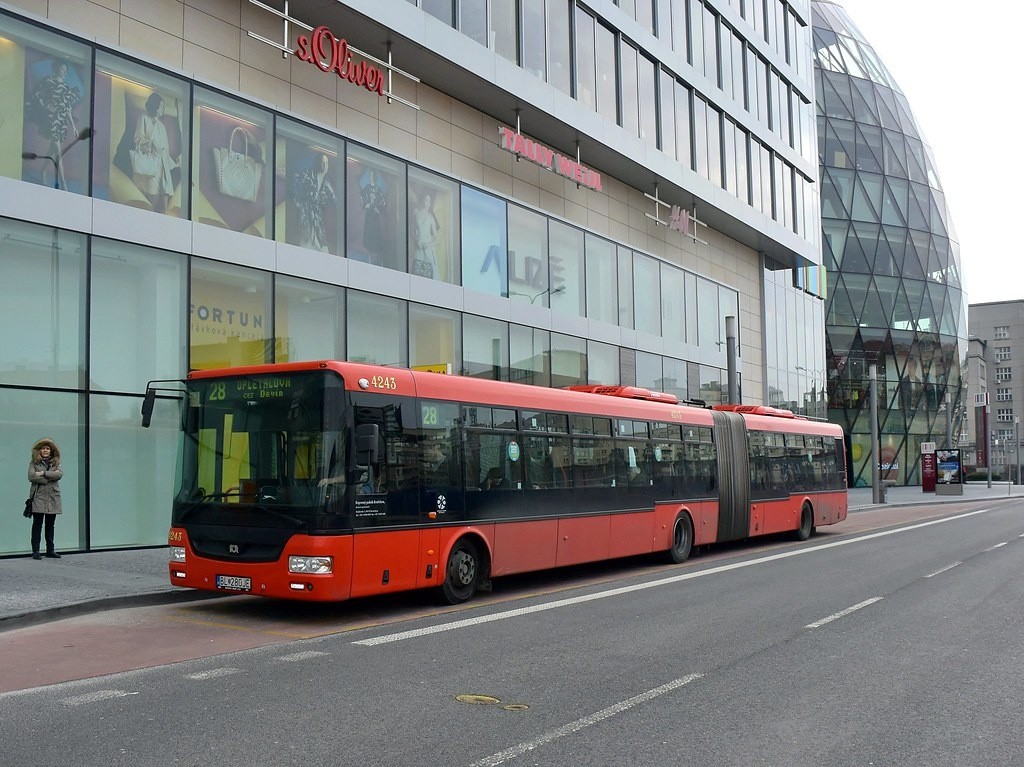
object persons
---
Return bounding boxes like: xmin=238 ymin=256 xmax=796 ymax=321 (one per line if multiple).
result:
xmin=480 ymin=466 xmax=509 ymax=490
xmin=317 ymin=458 xmax=373 ymax=514
xmin=898 ymin=358 xmax=921 ymax=410
xmin=34 ymin=60 xmax=80 ymax=191
xmin=28 ymin=438 xmax=62 ymax=560
xmin=288 ymin=153 xmax=336 ymax=253
xmin=410 ymin=192 xmax=438 ymax=279
xmin=427 ymin=448 xmax=448 ymax=484
xmin=359 ymin=168 xmax=387 ymax=264
xmin=133 ymin=93 xmax=176 ymax=214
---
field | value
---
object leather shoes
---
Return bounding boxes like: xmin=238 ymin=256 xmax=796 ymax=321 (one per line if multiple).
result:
xmin=32 ymin=550 xmax=42 ymax=560
xmin=45 ymin=550 xmax=62 ymax=559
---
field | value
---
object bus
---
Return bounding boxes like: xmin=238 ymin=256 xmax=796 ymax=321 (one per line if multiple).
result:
xmin=141 ymin=360 xmax=848 ymax=608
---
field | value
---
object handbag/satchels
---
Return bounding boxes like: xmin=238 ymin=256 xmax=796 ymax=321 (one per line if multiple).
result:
xmin=212 ymin=127 xmax=262 ymax=204
xmin=22 ymin=499 xmax=33 ymax=519
xmin=130 ymin=138 xmax=161 ymax=176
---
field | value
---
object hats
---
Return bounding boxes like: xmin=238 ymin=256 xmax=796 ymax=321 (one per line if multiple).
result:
xmin=487 ymin=467 xmax=503 ymax=478
